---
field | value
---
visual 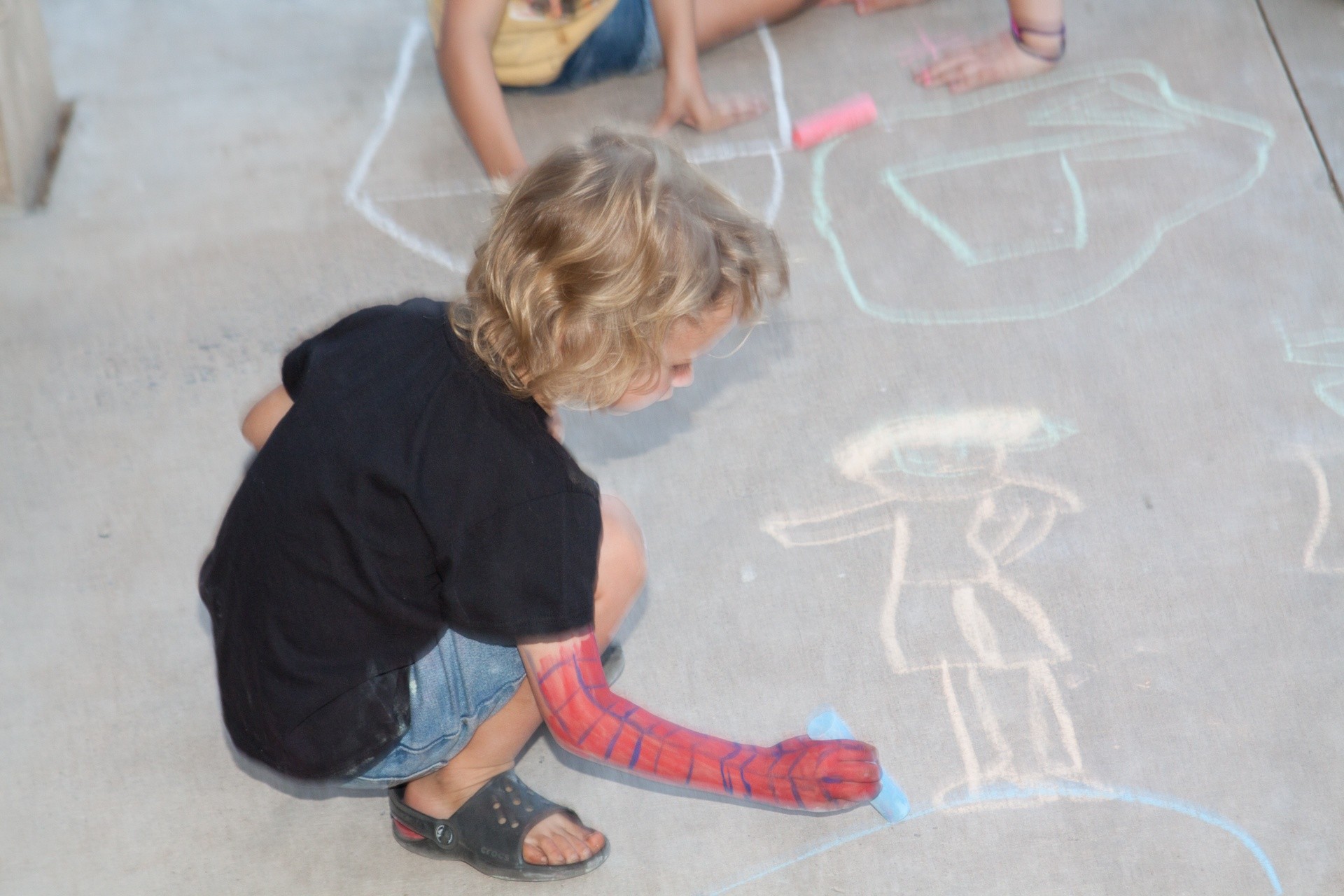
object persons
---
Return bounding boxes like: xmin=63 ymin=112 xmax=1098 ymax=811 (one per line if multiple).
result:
xmin=197 ymin=145 xmax=886 ymax=876
xmin=820 ymin=1 xmax=1064 ymax=97
xmin=429 ymin=0 xmax=812 ymax=214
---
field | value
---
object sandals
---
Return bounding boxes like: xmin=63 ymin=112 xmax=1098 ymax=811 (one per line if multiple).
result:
xmin=388 ymin=764 xmax=613 ymax=882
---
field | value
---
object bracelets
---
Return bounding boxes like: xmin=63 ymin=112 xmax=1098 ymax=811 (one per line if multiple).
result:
xmin=669 ymin=68 xmax=701 ymax=81
xmin=1009 ymin=17 xmax=1067 ymax=64
